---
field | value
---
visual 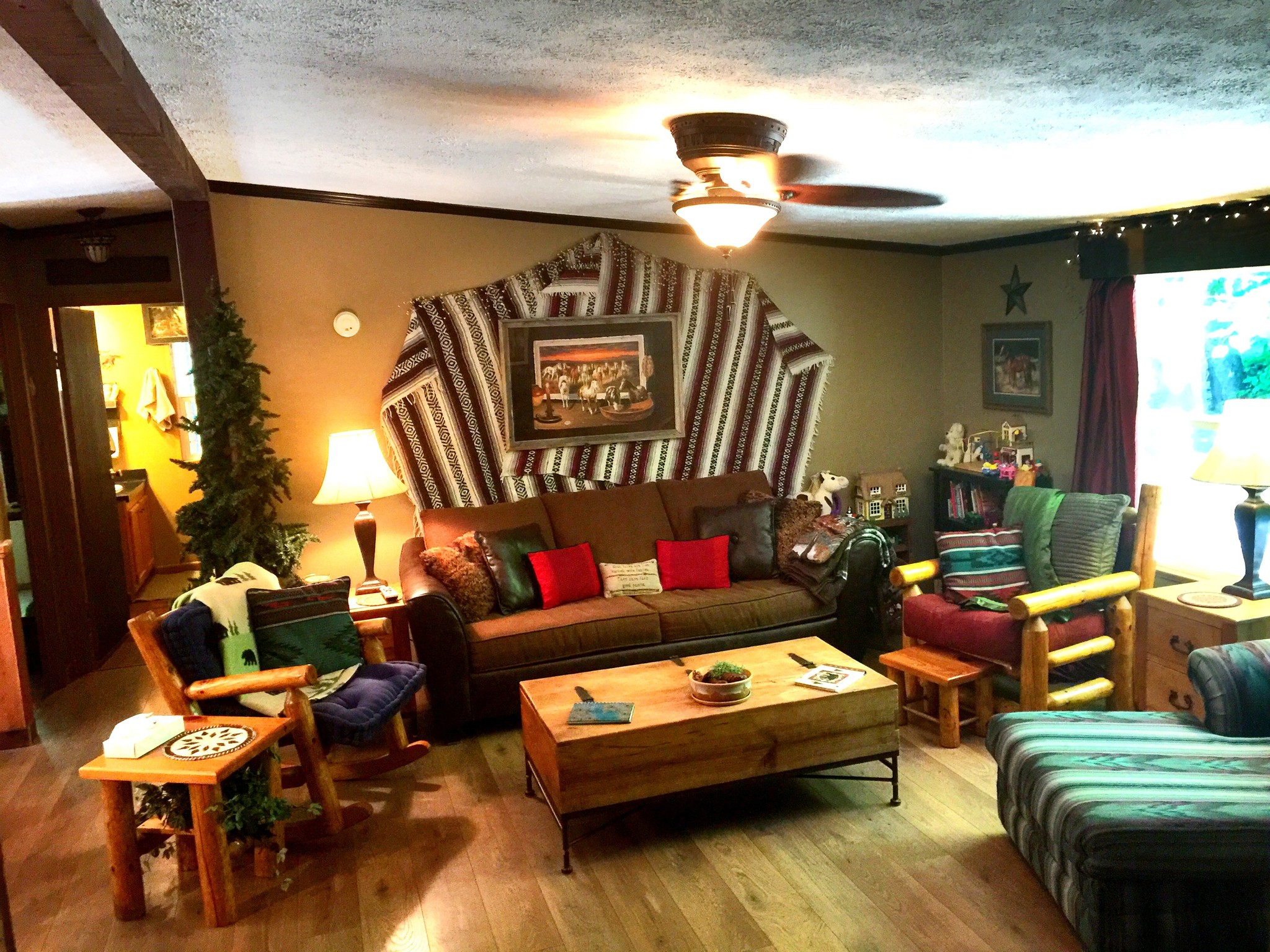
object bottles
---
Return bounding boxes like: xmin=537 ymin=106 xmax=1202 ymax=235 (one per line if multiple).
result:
xmin=1035 ymin=459 xmax=1043 ymax=469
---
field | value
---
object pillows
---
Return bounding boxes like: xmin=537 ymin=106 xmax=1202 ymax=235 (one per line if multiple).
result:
xmin=246 ymin=572 xmax=365 ymax=678
xmin=1046 ymin=486 xmax=1133 ymax=609
xmin=934 ymin=527 xmax=1034 ymax=603
xmin=160 ymin=601 xmax=255 ymax=716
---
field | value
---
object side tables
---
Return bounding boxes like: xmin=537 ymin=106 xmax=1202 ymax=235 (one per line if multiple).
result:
xmin=350 ymin=586 xmax=421 ymax=740
xmin=1128 ymin=576 xmax=1270 ymax=709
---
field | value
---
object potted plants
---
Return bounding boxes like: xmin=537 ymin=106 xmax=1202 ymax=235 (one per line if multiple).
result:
xmin=130 ymin=752 xmax=324 ymax=895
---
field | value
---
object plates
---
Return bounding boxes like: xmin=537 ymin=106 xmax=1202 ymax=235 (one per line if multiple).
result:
xmin=691 ymin=692 xmax=752 ymax=706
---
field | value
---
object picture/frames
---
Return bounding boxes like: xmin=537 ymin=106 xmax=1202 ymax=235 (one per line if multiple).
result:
xmin=143 ymin=301 xmax=189 ymax=344
xmin=496 ymin=310 xmax=686 ymax=452
xmin=981 ymin=319 xmax=1054 ymax=416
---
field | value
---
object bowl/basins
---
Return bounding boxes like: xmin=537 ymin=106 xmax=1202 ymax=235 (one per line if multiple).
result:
xmin=688 ymin=665 xmax=752 ymax=702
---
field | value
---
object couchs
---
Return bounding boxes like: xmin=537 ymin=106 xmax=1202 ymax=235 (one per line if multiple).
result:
xmin=977 ymin=632 xmax=1270 ymax=952
xmin=397 ymin=466 xmax=898 ymax=738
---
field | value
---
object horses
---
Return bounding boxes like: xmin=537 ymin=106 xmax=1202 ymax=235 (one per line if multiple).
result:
xmin=540 ymin=361 xmax=648 ymax=417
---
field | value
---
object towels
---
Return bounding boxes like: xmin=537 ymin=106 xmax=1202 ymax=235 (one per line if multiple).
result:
xmin=108 ymin=426 xmax=119 ymax=459
xmin=136 ymin=369 xmax=176 ymax=432
xmin=107 ymin=430 xmax=115 ymax=454
xmin=103 ymin=383 xmax=121 ymax=401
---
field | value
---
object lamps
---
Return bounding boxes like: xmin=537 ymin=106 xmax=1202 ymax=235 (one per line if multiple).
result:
xmin=667 ymin=112 xmax=790 ymax=323
xmin=312 ymin=429 xmax=410 ymax=598
xmin=1190 ymin=398 xmax=1270 ymax=601
xmin=77 ymin=206 xmax=114 ymax=269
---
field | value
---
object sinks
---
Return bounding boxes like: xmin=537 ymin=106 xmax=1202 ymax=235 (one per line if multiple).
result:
xmin=114 ymin=483 xmax=123 ymax=494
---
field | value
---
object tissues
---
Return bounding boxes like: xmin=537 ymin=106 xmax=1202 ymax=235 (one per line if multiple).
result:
xmin=101 ymin=712 xmax=184 ymax=759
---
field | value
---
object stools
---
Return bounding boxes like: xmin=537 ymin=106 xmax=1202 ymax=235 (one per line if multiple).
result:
xmin=878 ymin=642 xmax=997 ymax=750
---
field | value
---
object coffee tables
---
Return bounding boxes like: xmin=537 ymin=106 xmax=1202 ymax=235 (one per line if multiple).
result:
xmin=521 ymin=631 xmax=902 ymax=879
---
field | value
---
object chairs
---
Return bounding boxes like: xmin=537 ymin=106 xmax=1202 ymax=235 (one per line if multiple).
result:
xmin=896 ymin=481 xmax=1159 ymax=729
xmin=125 ymin=559 xmax=434 ymax=843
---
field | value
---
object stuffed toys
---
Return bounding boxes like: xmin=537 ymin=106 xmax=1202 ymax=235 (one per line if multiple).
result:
xmin=936 ymin=422 xmax=966 ymax=467
xmin=786 ymin=470 xmax=849 ymax=516
xmin=962 ymin=442 xmax=982 ymax=462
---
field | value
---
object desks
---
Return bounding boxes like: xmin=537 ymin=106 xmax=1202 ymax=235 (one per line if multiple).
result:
xmin=81 ymin=713 xmax=299 ymax=929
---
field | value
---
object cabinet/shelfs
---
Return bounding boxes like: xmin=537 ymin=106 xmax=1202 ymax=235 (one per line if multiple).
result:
xmin=116 ymin=488 xmax=155 ymax=605
xmin=932 ymin=458 xmax=1053 ymax=527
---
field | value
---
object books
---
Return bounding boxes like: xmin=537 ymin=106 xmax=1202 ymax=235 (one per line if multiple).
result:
xmin=794 ymin=662 xmax=868 ymax=693
xmin=566 ymin=702 xmax=636 ymax=722
